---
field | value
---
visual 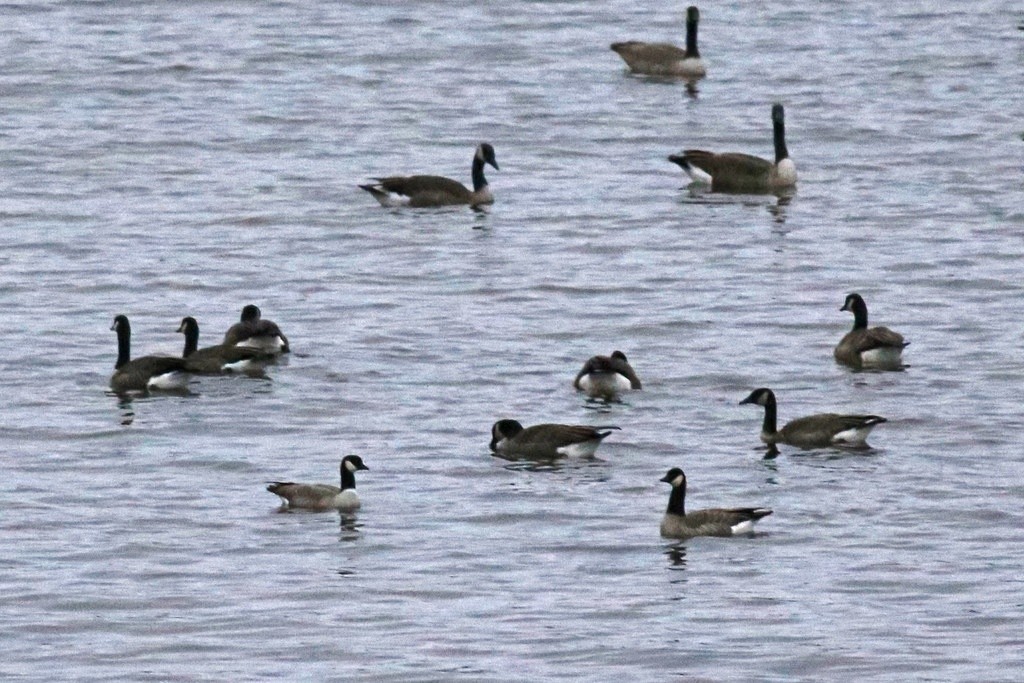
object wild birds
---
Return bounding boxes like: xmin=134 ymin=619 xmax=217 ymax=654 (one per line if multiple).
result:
xmin=574 ymin=350 xmax=641 ymax=391
xmin=609 ymin=6 xmax=708 ymax=76
xmin=667 ymin=104 xmax=800 ymax=188
xmin=174 ymin=317 xmax=267 ymax=373
xmin=90 ymin=302 xmax=206 ymax=393
xmin=224 ymin=306 xmax=288 ymax=352
xmin=491 ymin=420 xmax=622 ymax=460
xmin=659 ymin=467 xmax=773 ymax=540
xmin=833 ymin=293 xmax=911 ymax=369
xmin=264 ymin=455 xmax=369 ymax=510
xmin=357 ymin=143 xmax=500 ymax=207
xmin=739 ymin=388 xmax=888 ymax=445
